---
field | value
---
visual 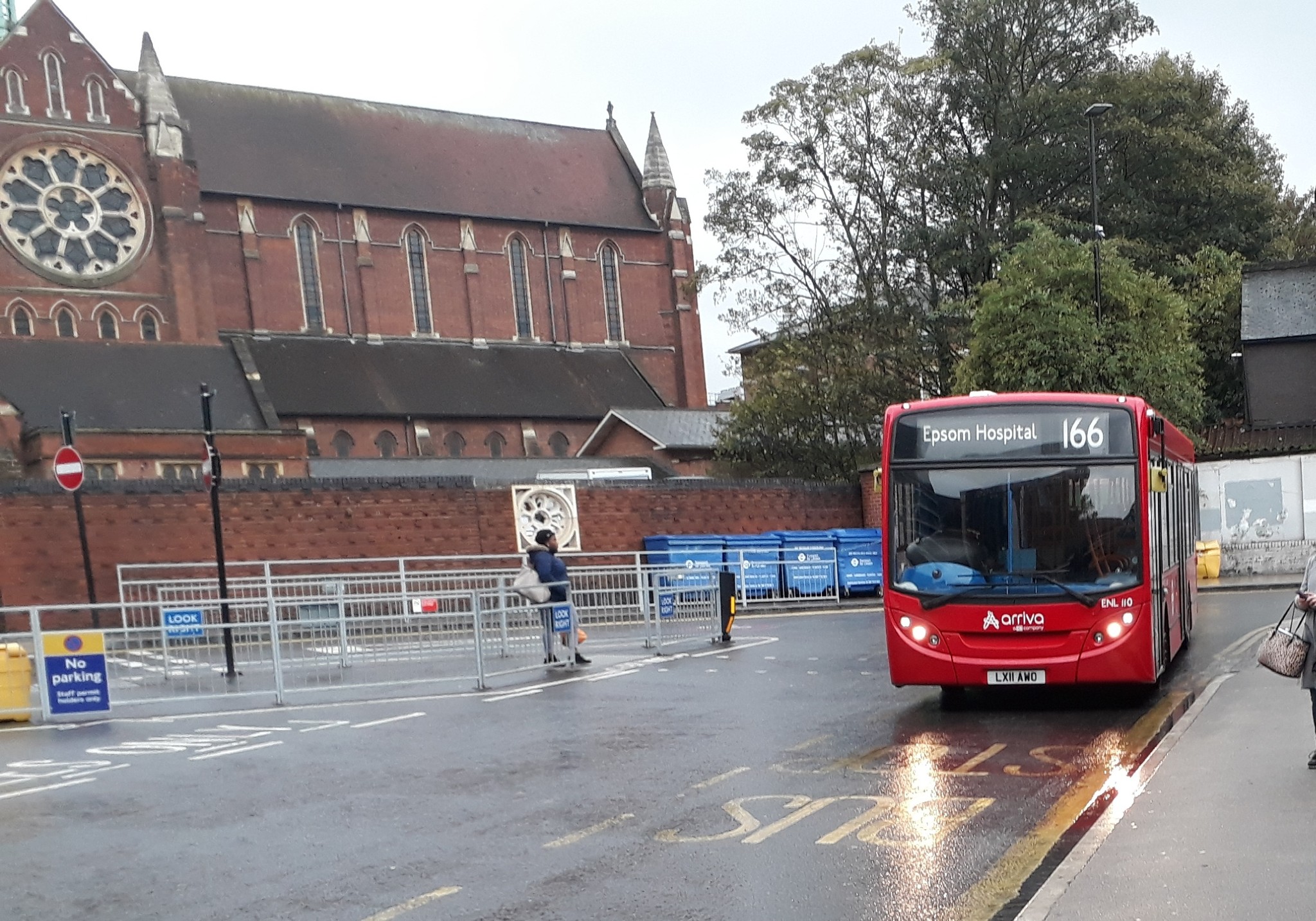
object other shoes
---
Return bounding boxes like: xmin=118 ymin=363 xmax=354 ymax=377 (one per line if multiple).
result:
xmin=1307 ymin=749 xmax=1316 ymax=769
xmin=544 ymin=654 xmax=566 ymax=667
xmin=569 ymin=653 xmax=591 ymax=663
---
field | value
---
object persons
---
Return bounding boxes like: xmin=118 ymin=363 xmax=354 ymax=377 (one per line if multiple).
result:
xmin=526 ymin=529 xmax=592 ymax=666
xmin=1293 ymin=550 xmax=1316 ymax=769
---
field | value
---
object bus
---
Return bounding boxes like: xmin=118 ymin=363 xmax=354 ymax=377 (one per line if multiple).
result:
xmin=873 ymin=390 xmax=1199 ymax=699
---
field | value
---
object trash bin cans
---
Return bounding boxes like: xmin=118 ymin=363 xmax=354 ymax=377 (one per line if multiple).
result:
xmin=1196 ymin=541 xmax=1221 ymax=579
xmin=642 ymin=534 xmax=726 ymax=599
xmin=830 ymin=527 xmax=883 ymax=598
xmin=772 ymin=530 xmax=837 ymax=597
xmin=722 ymin=533 xmax=782 ymax=597
xmin=0 ymin=642 xmax=30 ymax=722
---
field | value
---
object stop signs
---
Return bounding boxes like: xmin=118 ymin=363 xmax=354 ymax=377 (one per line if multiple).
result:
xmin=201 ymin=438 xmax=213 ymax=494
xmin=52 ymin=445 xmax=85 ymax=492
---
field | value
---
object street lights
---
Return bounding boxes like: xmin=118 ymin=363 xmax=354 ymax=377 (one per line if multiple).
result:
xmin=1084 ymin=103 xmax=1116 ymax=392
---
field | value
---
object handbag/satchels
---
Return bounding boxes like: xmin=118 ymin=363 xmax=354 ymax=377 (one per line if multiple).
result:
xmin=514 ymin=568 xmax=552 ymax=604
xmin=562 ymin=628 xmax=587 ymax=646
xmin=1256 ymin=599 xmax=1311 ymax=678
